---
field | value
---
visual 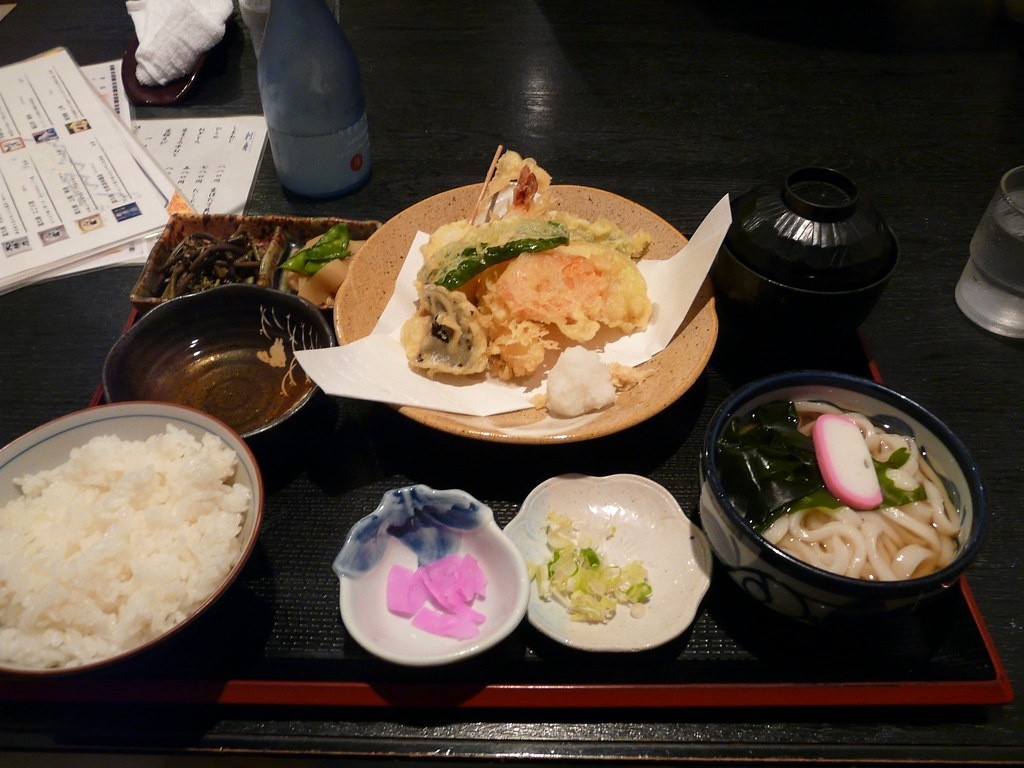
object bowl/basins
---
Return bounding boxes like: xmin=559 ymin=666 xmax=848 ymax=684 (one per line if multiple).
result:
xmin=334 ymin=183 xmax=718 ymax=446
xmin=713 ymin=164 xmax=902 ymax=350
xmin=332 ymin=484 xmax=530 ymax=668
xmin=103 ymin=284 xmax=333 ymax=439
xmin=699 ymin=372 xmax=986 ymax=624
xmin=503 ymin=472 xmax=713 ymax=654
xmin=0 ymin=401 xmax=265 ymax=674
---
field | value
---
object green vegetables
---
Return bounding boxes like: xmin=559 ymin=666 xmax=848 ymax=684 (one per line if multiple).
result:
xmin=152 ymin=225 xmax=265 ymax=300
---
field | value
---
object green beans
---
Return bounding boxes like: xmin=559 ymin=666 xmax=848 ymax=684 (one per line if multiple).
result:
xmin=279 ymin=221 xmax=350 ymax=276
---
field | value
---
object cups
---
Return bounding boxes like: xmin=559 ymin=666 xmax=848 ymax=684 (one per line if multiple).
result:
xmin=955 ymin=166 xmax=1024 ymax=341
xmin=239 ymin=0 xmax=339 ymax=58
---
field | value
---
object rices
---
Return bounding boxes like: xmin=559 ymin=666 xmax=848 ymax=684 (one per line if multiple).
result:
xmin=0 ymin=422 xmax=252 ymax=668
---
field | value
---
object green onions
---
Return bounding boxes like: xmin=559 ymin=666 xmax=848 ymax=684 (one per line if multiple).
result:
xmin=530 ymin=513 xmax=653 ymax=624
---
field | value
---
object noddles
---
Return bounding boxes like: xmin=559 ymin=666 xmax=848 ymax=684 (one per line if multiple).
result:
xmin=720 ymin=400 xmax=961 ymax=582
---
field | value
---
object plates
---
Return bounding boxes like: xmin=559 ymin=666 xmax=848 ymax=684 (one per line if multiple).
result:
xmin=130 ymin=211 xmax=383 ymax=313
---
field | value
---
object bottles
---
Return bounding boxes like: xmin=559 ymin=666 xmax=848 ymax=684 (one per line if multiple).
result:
xmin=257 ymin=0 xmax=372 ymax=200
